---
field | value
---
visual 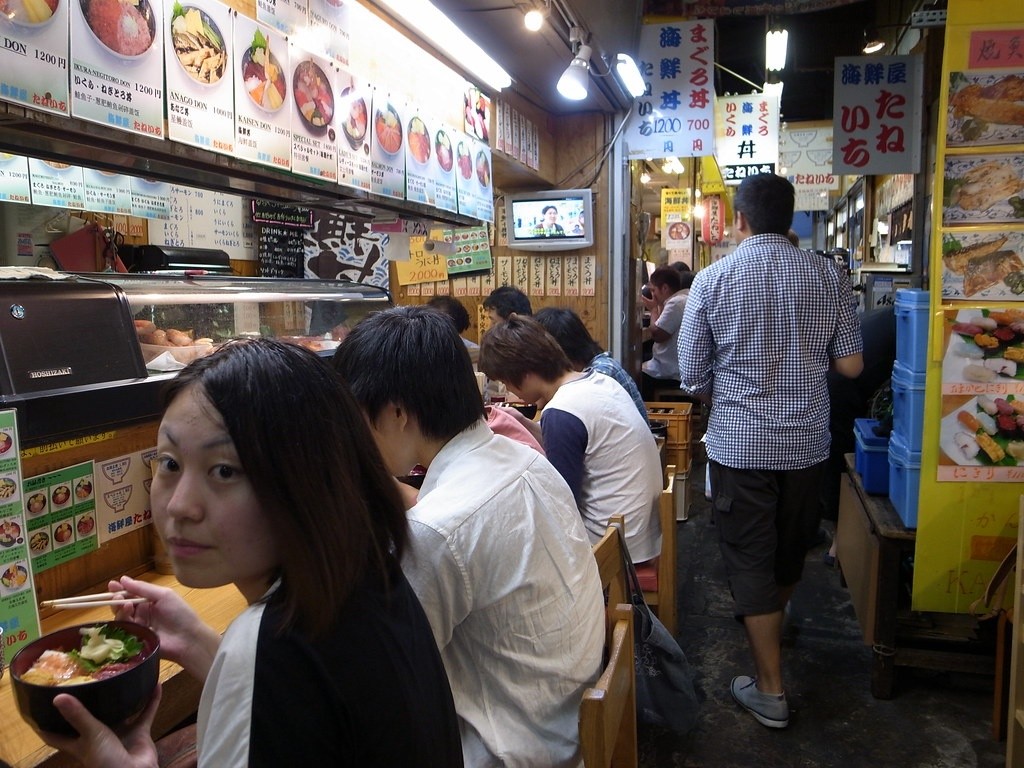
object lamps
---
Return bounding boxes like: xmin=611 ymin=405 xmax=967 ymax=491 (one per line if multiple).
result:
xmin=860 ymin=22 xmax=911 ymax=54
xmin=764 ymin=22 xmax=789 ymax=72
xmin=523 ymin=0 xmax=648 ymax=103
xmin=763 ymin=75 xmax=784 ymax=98
xmin=381 ymin=0 xmax=518 ymax=93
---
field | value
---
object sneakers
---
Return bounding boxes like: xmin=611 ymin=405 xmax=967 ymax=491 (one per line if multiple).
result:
xmin=781 ymin=602 xmax=791 ymax=642
xmin=730 ymin=675 xmax=789 ymax=728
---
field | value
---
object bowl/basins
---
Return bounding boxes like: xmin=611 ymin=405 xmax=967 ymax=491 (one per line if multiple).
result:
xmin=393 ymin=466 xmax=432 ymax=492
xmin=496 ymin=401 xmax=539 ymax=420
xmin=10 ymin=619 xmax=164 ymax=742
xmin=642 ymin=284 xmax=652 ymax=302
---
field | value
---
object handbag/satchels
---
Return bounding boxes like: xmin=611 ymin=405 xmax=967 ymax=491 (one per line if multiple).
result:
xmin=607 ymin=522 xmax=700 ymax=736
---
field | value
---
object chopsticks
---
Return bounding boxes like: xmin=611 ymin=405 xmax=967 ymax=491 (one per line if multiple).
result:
xmin=471 ymin=370 xmax=485 ymax=397
xmin=40 ymin=586 xmax=155 ymax=612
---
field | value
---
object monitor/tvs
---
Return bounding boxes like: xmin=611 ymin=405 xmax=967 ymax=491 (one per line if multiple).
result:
xmin=503 ymin=188 xmax=594 ymax=251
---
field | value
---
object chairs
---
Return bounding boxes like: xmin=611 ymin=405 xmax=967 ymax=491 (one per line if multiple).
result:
xmin=588 ymin=512 xmax=627 ymax=656
xmin=605 ymin=461 xmax=677 ymax=639
xmin=574 ymin=603 xmax=638 ymax=768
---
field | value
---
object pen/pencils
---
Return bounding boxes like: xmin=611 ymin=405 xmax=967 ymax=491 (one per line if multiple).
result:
xmin=155 ymin=270 xmax=208 ymax=275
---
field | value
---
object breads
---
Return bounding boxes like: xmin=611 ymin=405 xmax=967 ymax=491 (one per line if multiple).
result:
xmin=134 ymin=319 xmax=213 ymax=364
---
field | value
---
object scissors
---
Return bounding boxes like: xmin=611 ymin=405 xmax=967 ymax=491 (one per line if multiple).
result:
xmin=103 ymin=230 xmax=124 ymax=272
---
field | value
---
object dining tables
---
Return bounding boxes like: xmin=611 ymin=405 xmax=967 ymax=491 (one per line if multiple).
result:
xmin=0 ymin=563 xmax=248 ymax=768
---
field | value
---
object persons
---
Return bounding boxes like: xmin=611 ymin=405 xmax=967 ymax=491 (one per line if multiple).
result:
xmin=533 ymin=206 xmax=566 ymax=237
xmin=668 ymin=262 xmax=699 ymax=290
xmin=477 ymin=312 xmax=664 ymax=570
xmin=675 ymin=171 xmax=865 ymax=730
xmin=640 ymin=263 xmax=690 ymax=402
xmin=425 ymin=292 xmax=481 ymax=373
xmin=328 ymin=303 xmax=608 ymax=768
xmin=482 ymin=285 xmax=534 ymax=398
xmin=578 ymin=210 xmax=585 ymax=228
xmin=572 ymin=225 xmax=582 ymax=234
xmin=533 ymin=304 xmax=652 ymax=429
xmin=18 ymin=333 xmax=466 ymax=768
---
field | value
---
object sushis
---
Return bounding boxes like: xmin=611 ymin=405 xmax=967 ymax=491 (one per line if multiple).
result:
xmin=939 ymin=304 xmax=1024 ymax=470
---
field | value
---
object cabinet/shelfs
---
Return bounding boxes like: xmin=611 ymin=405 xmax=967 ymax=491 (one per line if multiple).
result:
xmin=0 ymin=263 xmax=399 ymax=451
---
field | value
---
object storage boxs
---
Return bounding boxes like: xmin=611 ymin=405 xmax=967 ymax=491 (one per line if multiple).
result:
xmin=887 ymin=429 xmax=922 ymax=528
xmin=890 ymin=360 xmax=927 ymax=452
xmin=894 ymin=286 xmax=930 ymax=372
xmin=853 ymin=418 xmax=889 ymax=495
xmin=643 ymin=400 xmax=694 ymax=474
xmin=674 ymin=470 xmax=692 ymax=521
